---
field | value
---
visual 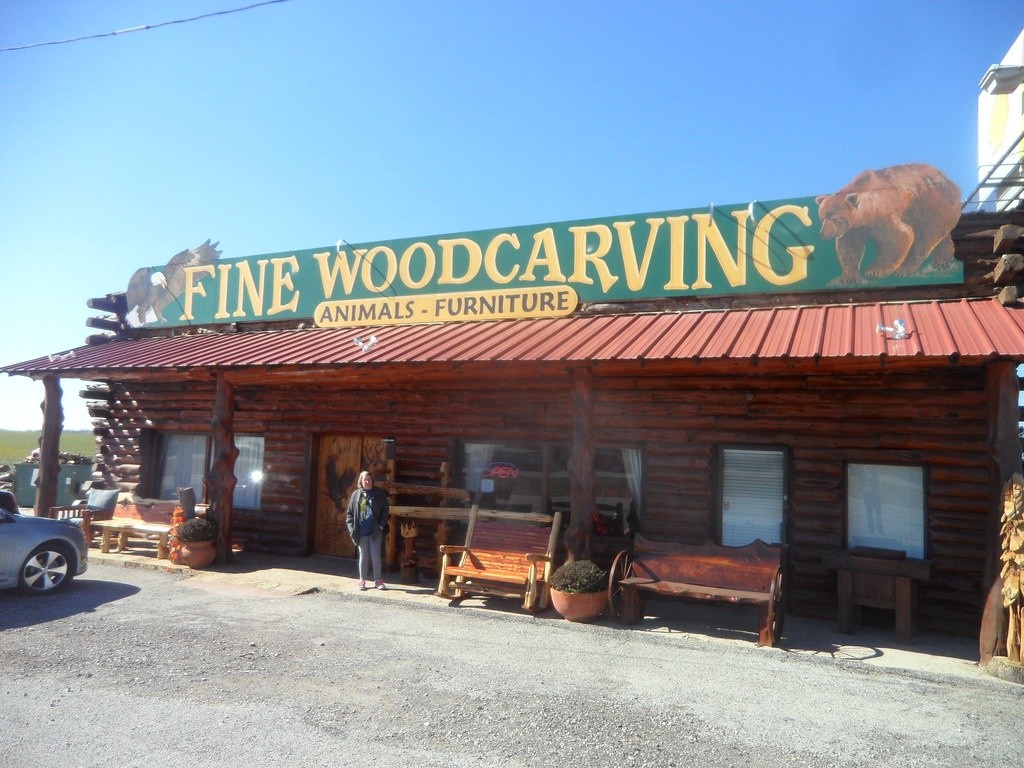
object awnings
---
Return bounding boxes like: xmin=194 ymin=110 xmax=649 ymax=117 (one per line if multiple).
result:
xmin=1 ymin=298 xmax=1024 ymax=666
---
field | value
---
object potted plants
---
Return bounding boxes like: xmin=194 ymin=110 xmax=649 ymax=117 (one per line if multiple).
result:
xmin=177 ymin=517 xmax=217 ymax=568
xmin=549 ymin=560 xmax=612 ymax=622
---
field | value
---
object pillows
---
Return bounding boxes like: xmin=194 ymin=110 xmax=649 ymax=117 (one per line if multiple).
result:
xmin=87 ymin=488 xmax=120 ymax=509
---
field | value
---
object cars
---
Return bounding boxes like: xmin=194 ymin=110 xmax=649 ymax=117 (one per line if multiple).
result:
xmin=0 ymin=507 xmax=88 ymax=595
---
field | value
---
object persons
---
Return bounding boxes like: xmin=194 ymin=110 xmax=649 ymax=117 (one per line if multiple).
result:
xmin=857 ymin=466 xmax=889 ymax=536
xmin=346 ymin=471 xmax=389 ymax=590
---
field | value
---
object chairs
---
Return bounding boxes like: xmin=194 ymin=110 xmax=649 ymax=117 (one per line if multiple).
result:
xmin=48 ymin=504 xmax=115 ymax=548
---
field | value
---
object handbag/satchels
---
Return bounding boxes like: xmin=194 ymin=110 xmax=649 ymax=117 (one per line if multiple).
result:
xmin=383 ymin=523 xmax=390 ymax=536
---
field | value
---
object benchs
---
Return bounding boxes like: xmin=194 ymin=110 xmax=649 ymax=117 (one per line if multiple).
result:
xmin=89 ymin=520 xmax=173 ymax=559
xmin=547 ymin=497 xmax=623 ymax=537
xmin=437 ymin=503 xmax=562 ymax=612
xmin=618 ymin=530 xmax=790 ymax=648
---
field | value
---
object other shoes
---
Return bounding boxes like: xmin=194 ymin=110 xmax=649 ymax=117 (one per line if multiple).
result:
xmin=358 ymin=580 xmax=367 ymax=591
xmin=374 ymin=578 xmax=386 ymax=590
